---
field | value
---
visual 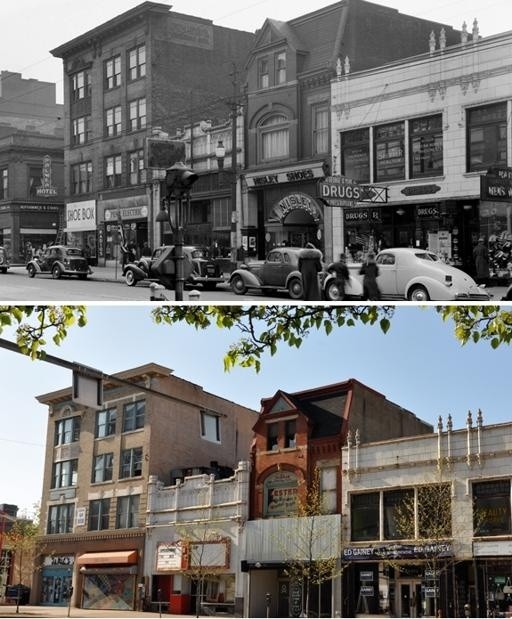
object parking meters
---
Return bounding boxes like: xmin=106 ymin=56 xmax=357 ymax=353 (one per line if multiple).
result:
xmin=264 ymin=592 xmax=273 ymax=618
xmin=156 ymin=587 xmax=162 ymax=618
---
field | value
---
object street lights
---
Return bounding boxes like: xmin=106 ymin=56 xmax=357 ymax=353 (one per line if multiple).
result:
xmin=156 ymin=160 xmax=200 ymax=302
xmin=216 ymin=138 xmax=237 ymax=252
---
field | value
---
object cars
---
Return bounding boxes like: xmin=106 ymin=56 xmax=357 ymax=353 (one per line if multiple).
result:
xmin=0 ymin=246 xmax=10 ymax=274
xmin=123 ymin=245 xmax=225 ymax=287
xmin=228 ymin=247 xmax=327 ymax=298
xmin=26 ymin=245 xmax=93 ymax=280
xmin=322 ymin=247 xmax=491 ymax=301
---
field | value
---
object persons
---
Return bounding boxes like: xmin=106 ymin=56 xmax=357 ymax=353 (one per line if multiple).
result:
xmin=359 ymin=255 xmax=381 ymax=301
xmin=327 ymin=254 xmax=352 ymax=300
xmin=82 ymin=245 xmax=94 ymax=274
xmin=126 ymin=239 xmax=152 ymax=263
xmin=298 ymin=242 xmax=323 ymax=301
xmin=25 ymin=243 xmax=50 ymax=261
xmin=472 ymin=238 xmax=491 ymax=287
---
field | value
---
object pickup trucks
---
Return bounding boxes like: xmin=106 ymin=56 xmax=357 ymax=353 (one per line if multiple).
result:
xmin=6 ymin=584 xmax=31 ymax=605
